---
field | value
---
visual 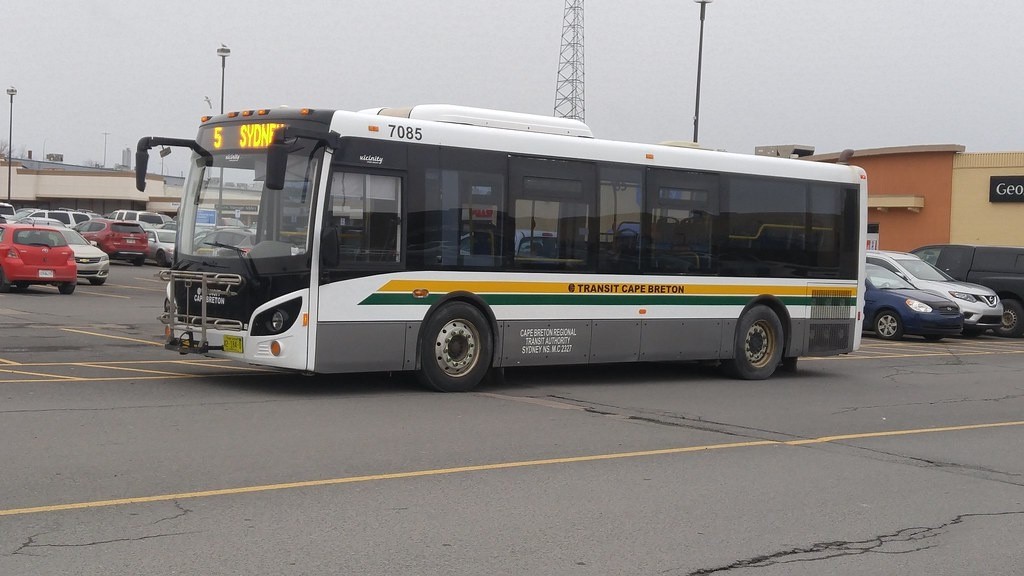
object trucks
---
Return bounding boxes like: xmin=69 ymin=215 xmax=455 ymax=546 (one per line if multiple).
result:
xmin=905 ymin=243 xmax=1024 ymax=338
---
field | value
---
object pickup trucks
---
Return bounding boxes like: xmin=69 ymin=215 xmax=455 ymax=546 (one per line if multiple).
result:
xmin=108 ymin=210 xmax=175 ymax=229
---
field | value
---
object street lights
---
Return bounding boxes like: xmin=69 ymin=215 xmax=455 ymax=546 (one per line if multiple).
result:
xmin=103 ymin=132 xmax=110 ymax=168
xmin=216 ymin=41 xmax=232 ymax=115
xmin=6 ymin=85 xmax=19 ymax=199
xmin=692 ymin=0 xmax=713 ymax=143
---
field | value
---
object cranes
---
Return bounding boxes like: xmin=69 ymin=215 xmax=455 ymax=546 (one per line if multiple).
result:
xmin=551 ymin=0 xmax=587 ymax=122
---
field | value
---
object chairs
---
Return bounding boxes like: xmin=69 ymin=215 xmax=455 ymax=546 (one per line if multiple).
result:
xmin=368 ymin=208 xmax=840 ymax=275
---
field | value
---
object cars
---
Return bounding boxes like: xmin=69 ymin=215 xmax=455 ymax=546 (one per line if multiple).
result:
xmin=218 ymin=214 xmax=250 ymax=230
xmin=52 ymin=227 xmax=110 ymax=285
xmin=865 ymin=250 xmax=1004 ymax=337
xmin=161 ymin=222 xmax=177 ymax=230
xmin=59 ymin=207 xmax=95 ymax=212
xmin=16 ymin=207 xmax=41 ymax=213
xmin=864 ymin=263 xmax=964 ymax=341
xmin=0 ymin=201 xmax=16 ymax=223
xmin=194 ymin=230 xmax=257 ymax=255
xmin=0 ymin=223 xmax=78 ymax=295
xmin=72 ymin=218 xmax=150 ymax=266
xmin=88 ymin=212 xmax=103 ymax=218
xmin=12 ymin=217 xmax=66 ymax=226
xmin=19 ymin=211 xmax=92 ymax=228
xmin=143 ymin=228 xmax=177 ymax=267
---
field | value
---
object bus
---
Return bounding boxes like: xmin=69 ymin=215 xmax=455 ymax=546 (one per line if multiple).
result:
xmin=136 ymin=102 xmax=868 ymax=393
